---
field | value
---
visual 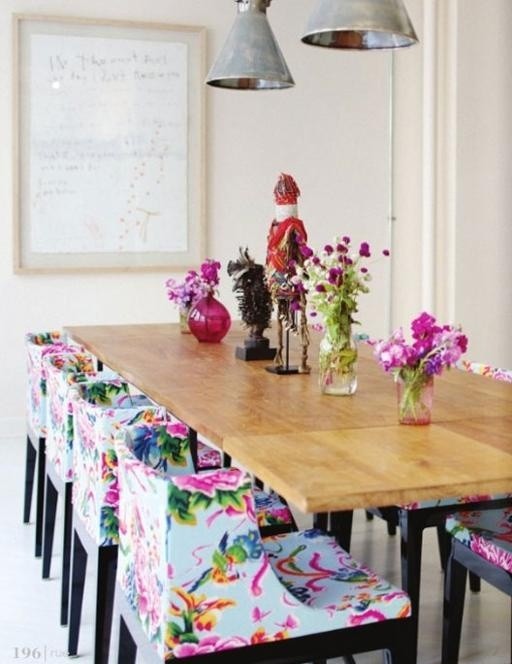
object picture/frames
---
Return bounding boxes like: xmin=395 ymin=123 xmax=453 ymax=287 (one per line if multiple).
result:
xmin=10 ymin=12 xmax=209 ymax=276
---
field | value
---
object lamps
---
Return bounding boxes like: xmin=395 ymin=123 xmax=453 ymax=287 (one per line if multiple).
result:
xmin=299 ymin=0 xmax=418 ymax=50
xmin=204 ymin=0 xmax=294 ymax=89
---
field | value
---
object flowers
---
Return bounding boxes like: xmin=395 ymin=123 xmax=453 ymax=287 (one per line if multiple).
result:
xmin=166 ymin=257 xmax=221 ymax=306
xmin=302 ymin=235 xmax=390 ymax=397
xmin=371 ymin=314 xmax=468 ymax=424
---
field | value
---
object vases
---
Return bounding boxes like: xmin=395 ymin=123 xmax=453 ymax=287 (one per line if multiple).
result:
xmin=187 ymin=294 xmax=232 ymax=343
xmin=178 ymin=305 xmax=192 ymax=334
